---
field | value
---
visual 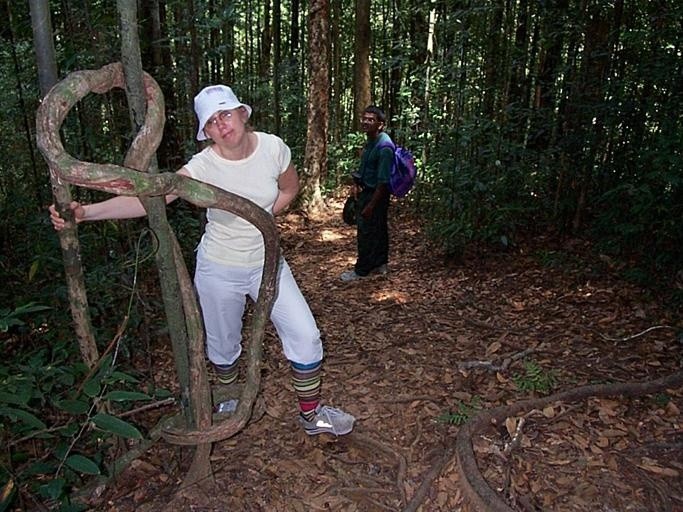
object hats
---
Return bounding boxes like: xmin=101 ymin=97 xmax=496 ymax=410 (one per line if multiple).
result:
xmin=193 ymin=83 xmax=253 ymax=142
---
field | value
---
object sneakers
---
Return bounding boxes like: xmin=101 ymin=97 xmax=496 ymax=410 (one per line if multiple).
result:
xmin=338 ymin=264 xmax=387 ymax=283
xmin=297 ymin=402 xmax=357 ymax=439
xmin=216 ymin=398 xmax=239 ymax=413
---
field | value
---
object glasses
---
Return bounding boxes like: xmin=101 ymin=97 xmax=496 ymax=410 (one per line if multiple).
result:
xmin=204 ymin=111 xmax=233 ymax=131
xmin=360 ymin=118 xmax=376 ymax=123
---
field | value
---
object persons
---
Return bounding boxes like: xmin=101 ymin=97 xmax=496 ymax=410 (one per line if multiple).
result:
xmin=337 ymin=103 xmax=393 ymax=286
xmin=45 ymin=82 xmax=358 ymax=438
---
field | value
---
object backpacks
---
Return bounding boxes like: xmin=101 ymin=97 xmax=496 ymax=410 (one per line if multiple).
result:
xmin=373 ymin=141 xmax=418 ymax=197
